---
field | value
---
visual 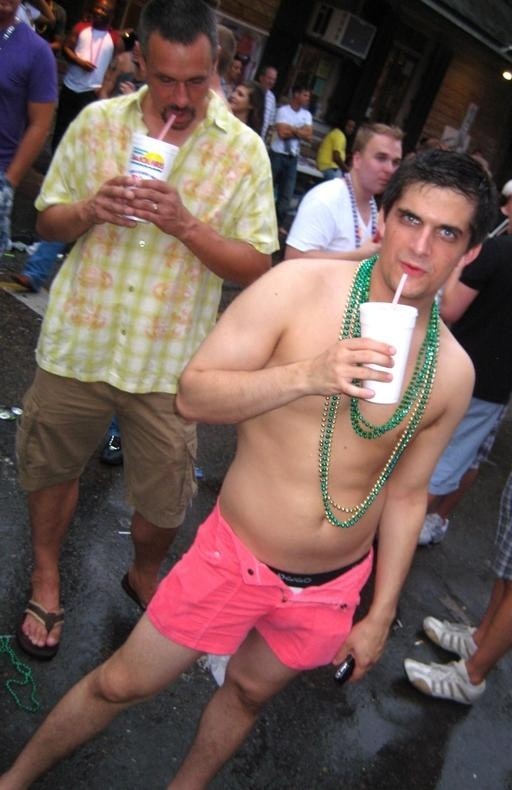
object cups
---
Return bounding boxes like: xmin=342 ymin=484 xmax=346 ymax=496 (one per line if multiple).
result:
xmin=357 ymin=300 xmax=419 ymax=406
xmin=115 ymin=131 xmax=181 ymax=226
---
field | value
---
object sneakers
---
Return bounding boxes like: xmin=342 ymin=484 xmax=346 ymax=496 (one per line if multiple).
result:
xmin=101 ymin=436 xmax=122 ymax=464
xmin=418 ymin=513 xmax=448 ymax=545
xmin=423 ymin=615 xmax=477 ymax=660
xmin=404 ymin=658 xmax=485 ymax=705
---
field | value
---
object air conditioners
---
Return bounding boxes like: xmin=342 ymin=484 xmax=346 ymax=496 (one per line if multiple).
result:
xmin=306 ymin=2 xmax=377 ymax=63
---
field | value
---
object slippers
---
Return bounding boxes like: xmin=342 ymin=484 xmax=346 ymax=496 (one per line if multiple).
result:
xmin=18 ymin=600 xmax=64 ymax=660
xmin=122 ymin=573 xmax=147 ymax=612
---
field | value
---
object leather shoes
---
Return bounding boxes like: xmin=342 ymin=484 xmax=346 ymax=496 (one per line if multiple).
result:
xmin=11 ymin=275 xmax=38 ymax=292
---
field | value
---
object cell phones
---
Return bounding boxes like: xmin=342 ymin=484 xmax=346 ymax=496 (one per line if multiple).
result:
xmin=335 ymin=655 xmax=354 ymax=681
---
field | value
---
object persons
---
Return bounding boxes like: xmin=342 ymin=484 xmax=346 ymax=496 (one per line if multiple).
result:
xmin=0 ymin=0 xmax=512 ymax=790
